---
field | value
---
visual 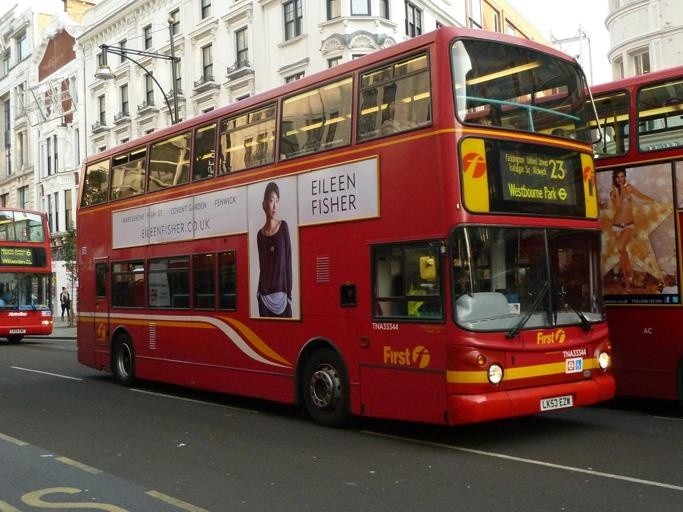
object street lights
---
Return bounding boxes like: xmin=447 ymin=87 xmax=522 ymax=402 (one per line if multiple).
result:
xmin=95 ymin=18 xmax=182 ymax=125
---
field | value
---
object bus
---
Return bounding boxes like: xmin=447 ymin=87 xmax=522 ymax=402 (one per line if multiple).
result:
xmin=0 ymin=207 xmax=55 ymax=345
xmin=74 ymin=25 xmax=617 ymax=428
xmin=465 ymin=65 xmax=683 ymax=400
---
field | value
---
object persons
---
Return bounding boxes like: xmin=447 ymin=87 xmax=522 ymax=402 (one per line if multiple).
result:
xmin=379 ymin=100 xmax=421 ymax=135
xmin=60 ymin=287 xmax=71 ymax=323
xmin=255 ymin=181 xmax=295 ymax=318
xmin=0 ymin=291 xmax=6 ymax=306
xmin=557 ymin=251 xmax=588 ymax=310
xmin=606 ymin=164 xmax=657 ymax=295
